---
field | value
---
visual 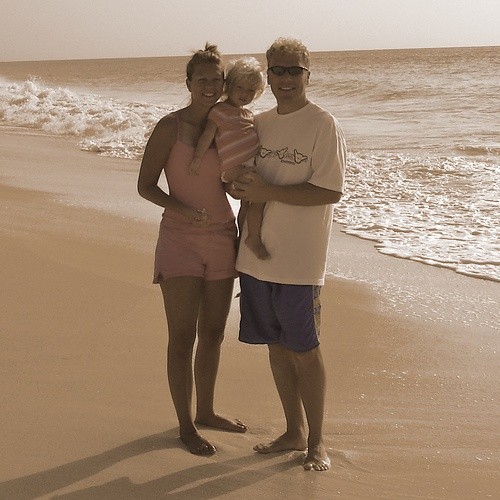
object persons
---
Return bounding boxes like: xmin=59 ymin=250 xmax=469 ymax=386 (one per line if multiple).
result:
xmin=187 ymin=56 xmax=272 ymax=261
xmin=223 ymin=35 xmax=348 ymax=472
xmin=137 ymin=41 xmax=248 ymax=457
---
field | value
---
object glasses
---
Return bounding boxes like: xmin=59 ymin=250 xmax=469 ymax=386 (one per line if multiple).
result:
xmin=268 ymin=66 xmax=309 ymax=76
